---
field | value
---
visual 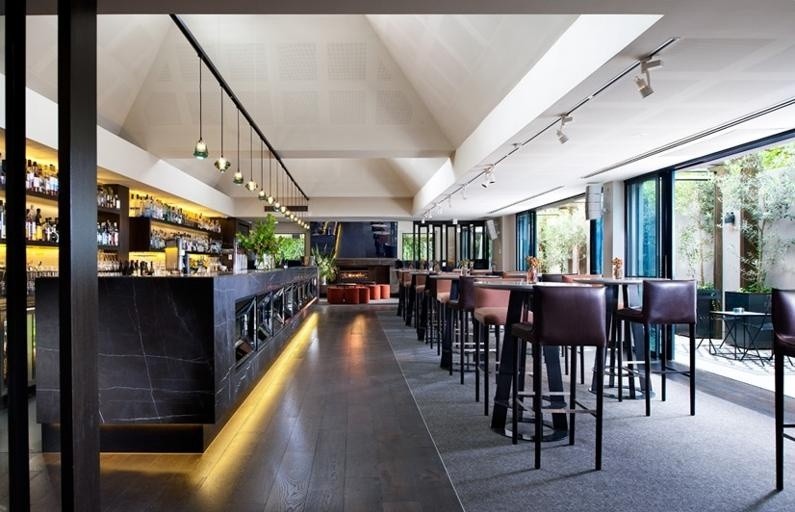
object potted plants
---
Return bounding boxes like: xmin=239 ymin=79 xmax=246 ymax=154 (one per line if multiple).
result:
xmin=723 ymin=151 xmax=795 ymax=349
xmin=235 ymin=214 xmax=279 ymax=271
xmin=276 ymin=236 xmax=305 ymax=269
xmin=674 ymin=174 xmax=728 ymax=341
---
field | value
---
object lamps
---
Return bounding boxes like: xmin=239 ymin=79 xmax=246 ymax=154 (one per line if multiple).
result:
xmin=193 ymin=50 xmax=312 ymax=234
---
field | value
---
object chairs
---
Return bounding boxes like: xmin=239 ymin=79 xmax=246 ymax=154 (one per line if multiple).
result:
xmin=770 ymin=286 xmax=795 ymax=492
xmin=696 ymin=286 xmax=794 ymax=368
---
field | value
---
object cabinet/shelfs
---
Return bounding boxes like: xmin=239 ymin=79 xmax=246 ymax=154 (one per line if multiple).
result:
xmin=1 ymin=155 xmax=133 ymax=401
xmin=130 ymin=216 xmax=225 ymax=256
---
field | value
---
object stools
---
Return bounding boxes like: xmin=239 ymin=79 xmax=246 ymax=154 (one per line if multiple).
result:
xmin=326 ymin=281 xmax=391 ymax=305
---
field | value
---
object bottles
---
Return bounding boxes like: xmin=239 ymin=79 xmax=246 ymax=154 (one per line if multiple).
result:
xmin=0 ymin=151 xmax=121 ymax=209
xmin=0 ymin=198 xmax=119 ymax=248
xmin=129 ymin=193 xmax=221 ymax=233
xmin=0 ymin=264 xmax=58 ymax=295
xmin=96 ymin=253 xmax=121 ymax=272
xmin=187 ymin=256 xmax=222 ymax=272
xmin=122 ymin=259 xmax=155 ymax=276
xmin=149 ymin=228 xmax=225 ymax=255
